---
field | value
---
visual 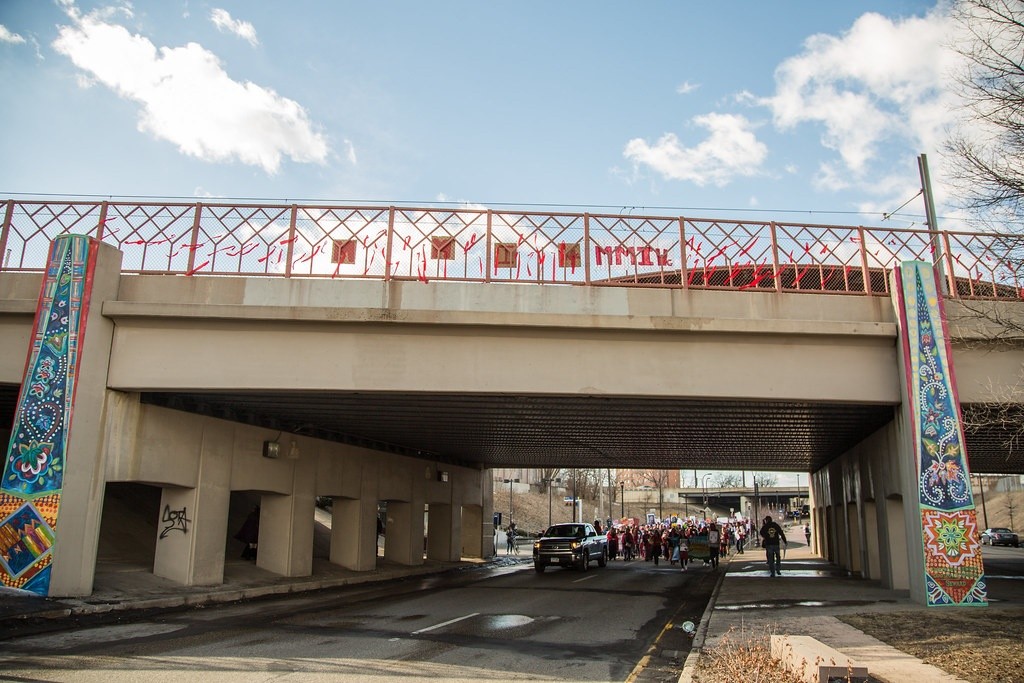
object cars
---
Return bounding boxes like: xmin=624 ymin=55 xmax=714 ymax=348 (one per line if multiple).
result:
xmin=980 ymin=528 xmax=1019 ymax=548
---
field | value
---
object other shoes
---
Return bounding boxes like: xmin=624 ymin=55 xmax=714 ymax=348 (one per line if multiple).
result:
xmin=624 ymin=558 xmax=627 ymax=562
xmin=703 ymin=563 xmax=709 ymax=567
xmin=684 ymin=566 xmax=687 ymax=570
xmin=681 ymin=568 xmax=684 ymax=571
xmin=770 ymin=574 xmax=775 ymax=578
xmin=777 ymin=571 xmax=781 ymax=575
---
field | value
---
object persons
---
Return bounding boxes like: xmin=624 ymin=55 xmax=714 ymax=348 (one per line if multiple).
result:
xmin=593 ymin=517 xmax=754 ymax=572
xmin=803 ymin=523 xmax=811 ymax=546
xmin=506 ymin=523 xmax=518 ymax=556
xmin=760 ymin=516 xmax=788 ymax=577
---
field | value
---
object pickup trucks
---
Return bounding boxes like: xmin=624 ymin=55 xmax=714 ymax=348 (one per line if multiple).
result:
xmin=533 ymin=523 xmax=610 ymax=571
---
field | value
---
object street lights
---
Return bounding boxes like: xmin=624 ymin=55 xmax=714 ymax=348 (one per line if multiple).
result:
xmin=503 ymin=479 xmax=520 ymax=526
xmin=701 ymin=473 xmax=710 ymax=520
xmin=541 ymin=478 xmax=561 ymax=528
xmin=620 ymin=481 xmax=625 ymax=518
